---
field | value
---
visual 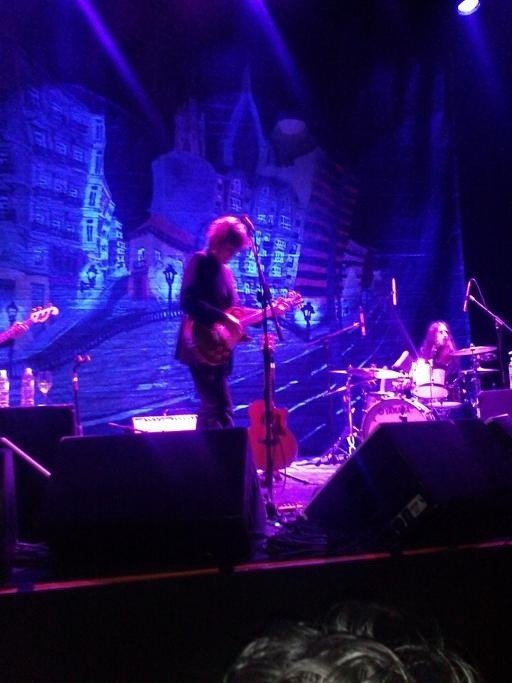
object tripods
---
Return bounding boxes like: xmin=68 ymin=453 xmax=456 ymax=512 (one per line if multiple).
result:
xmin=315 ymin=384 xmax=361 ymax=466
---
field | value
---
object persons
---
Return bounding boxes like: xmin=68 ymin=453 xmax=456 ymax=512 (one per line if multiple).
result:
xmin=0 ymin=320 xmax=30 ymax=349
xmin=174 ymin=217 xmax=291 ymax=430
xmin=417 ymin=321 xmax=460 ymax=384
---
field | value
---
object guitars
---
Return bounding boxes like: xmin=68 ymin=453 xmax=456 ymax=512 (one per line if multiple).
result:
xmin=1 ymin=302 xmax=59 ymax=344
xmin=248 ymin=332 xmax=296 ymax=471
xmin=181 ymin=291 xmax=304 ymax=367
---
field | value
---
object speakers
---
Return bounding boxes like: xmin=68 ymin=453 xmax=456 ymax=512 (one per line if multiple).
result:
xmin=57 ymin=426 xmax=273 ymax=568
xmin=0 ymin=403 xmax=76 ymax=545
xmin=302 ymin=419 xmax=512 ymax=548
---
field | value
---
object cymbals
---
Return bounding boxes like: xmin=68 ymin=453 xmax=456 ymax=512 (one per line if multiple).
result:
xmin=460 ymin=368 xmax=497 ymax=374
xmin=331 ymin=370 xmax=348 ymax=375
xmin=447 ymin=346 xmax=496 ymax=355
xmin=345 ymin=367 xmax=403 ymax=379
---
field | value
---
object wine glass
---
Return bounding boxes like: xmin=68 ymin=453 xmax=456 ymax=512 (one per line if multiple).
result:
xmin=36 ymin=371 xmax=52 ymax=406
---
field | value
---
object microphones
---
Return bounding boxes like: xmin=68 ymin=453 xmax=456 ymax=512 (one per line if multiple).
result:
xmin=392 ymin=278 xmax=397 ymax=305
xmin=463 ymin=279 xmax=473 ymax=312
xmin=359 ymin=307 xmax=366 ymax=336
xmin=242 ymin=213 xmax=255 ymax=234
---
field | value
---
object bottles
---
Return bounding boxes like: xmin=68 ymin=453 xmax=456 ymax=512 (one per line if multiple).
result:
xmin=0 ymin=369 xmax=10 ymax=407
xmin=20 ymin=367 xmax=35 ymax=406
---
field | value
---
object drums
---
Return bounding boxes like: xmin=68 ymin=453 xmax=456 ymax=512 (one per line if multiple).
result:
xmin=411 ymin=360 xmax=449 ymax=399
xmin=362 ymin=391 xmax=405 ymax=409
xmin=427 ymin=402 xmax=462 ymax=419
xmin=361 ymin=397 xmax=435 ymax=445
